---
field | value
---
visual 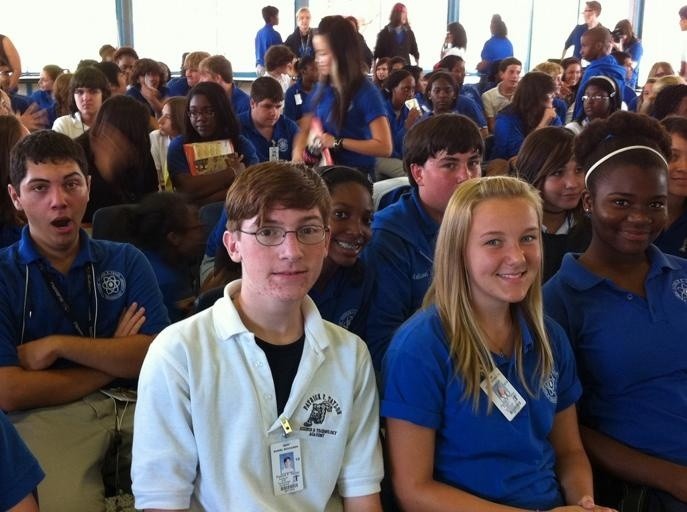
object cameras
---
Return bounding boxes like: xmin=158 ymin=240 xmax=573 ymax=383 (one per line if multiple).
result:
xmin=609 ymin=28 xmax=622 ymax=44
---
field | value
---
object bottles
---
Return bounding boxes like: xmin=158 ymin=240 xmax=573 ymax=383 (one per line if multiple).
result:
xmin=299 ymin=136 xmax=321 ymax=168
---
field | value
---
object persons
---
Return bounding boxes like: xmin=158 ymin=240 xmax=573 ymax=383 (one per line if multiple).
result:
xmin=1 ymin=0 xmax=687 ymax=512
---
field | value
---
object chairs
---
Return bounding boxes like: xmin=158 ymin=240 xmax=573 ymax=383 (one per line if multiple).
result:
xmin=197 ymin=200 xmax=222 ymax=236
xmin=91 ymin=204 xmax=140 ymax=243
xmin=373 ymin=175 xmax=412 ymax=209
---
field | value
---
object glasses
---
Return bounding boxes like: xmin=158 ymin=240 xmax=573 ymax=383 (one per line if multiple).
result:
xmin=186 ymin=108 xmax=216 ymax=120
xmin=235 ymin=223 xmax=329 ymax=247
xmin=580 ymin=92 xmax=617 ymax=105
